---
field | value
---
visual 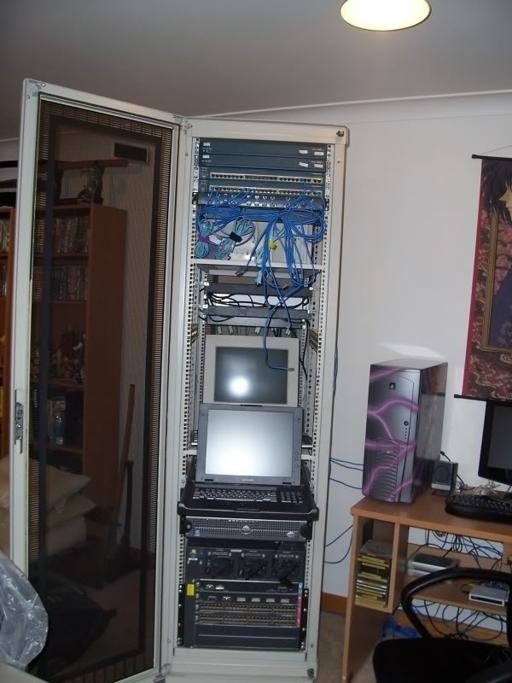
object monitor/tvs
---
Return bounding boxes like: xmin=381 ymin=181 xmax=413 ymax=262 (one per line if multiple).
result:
xmin=195 ymin=404 xmax=303 ymax=485
xmin=205 ymin=334 xmax=299 ymax=406
xmin=478 ymin=398 xmax=512 ymax=486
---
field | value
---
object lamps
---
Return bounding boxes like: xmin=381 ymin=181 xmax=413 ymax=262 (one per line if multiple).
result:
xmin=339 ymin=0 xmax=433 ymax=33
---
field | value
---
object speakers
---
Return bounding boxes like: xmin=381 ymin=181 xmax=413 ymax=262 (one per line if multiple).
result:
xmin=431 ymin=460 xmax=458 ymax=491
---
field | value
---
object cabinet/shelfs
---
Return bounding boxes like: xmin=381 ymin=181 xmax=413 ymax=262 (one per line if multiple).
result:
xmin=32 ymin=201 xmax=127 ymax=515
xmin=1 ymin=206 xmax=17 ymax=468
xmin=332 ymin=491 xmax=512 ymax=682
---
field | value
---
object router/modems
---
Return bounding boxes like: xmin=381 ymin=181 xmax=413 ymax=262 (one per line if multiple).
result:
xmin=468 ymin=584 xmax=507 ymax=607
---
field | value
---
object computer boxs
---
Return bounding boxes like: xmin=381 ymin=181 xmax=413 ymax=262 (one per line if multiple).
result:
xmin=364 ymin=355 xmax=448 ymax=503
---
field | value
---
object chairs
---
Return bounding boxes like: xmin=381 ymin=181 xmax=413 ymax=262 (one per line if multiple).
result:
xmin=367 ymin=566 xmax=512 ymax=683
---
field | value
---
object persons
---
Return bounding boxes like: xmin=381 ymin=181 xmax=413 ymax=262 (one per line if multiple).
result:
xmin=482 ymin=158 xmax=512 ymax=351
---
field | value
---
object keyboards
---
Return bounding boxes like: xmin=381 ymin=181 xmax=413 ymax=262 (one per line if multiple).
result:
xmin=445 ymin=490 xmax=512 ymax=523
